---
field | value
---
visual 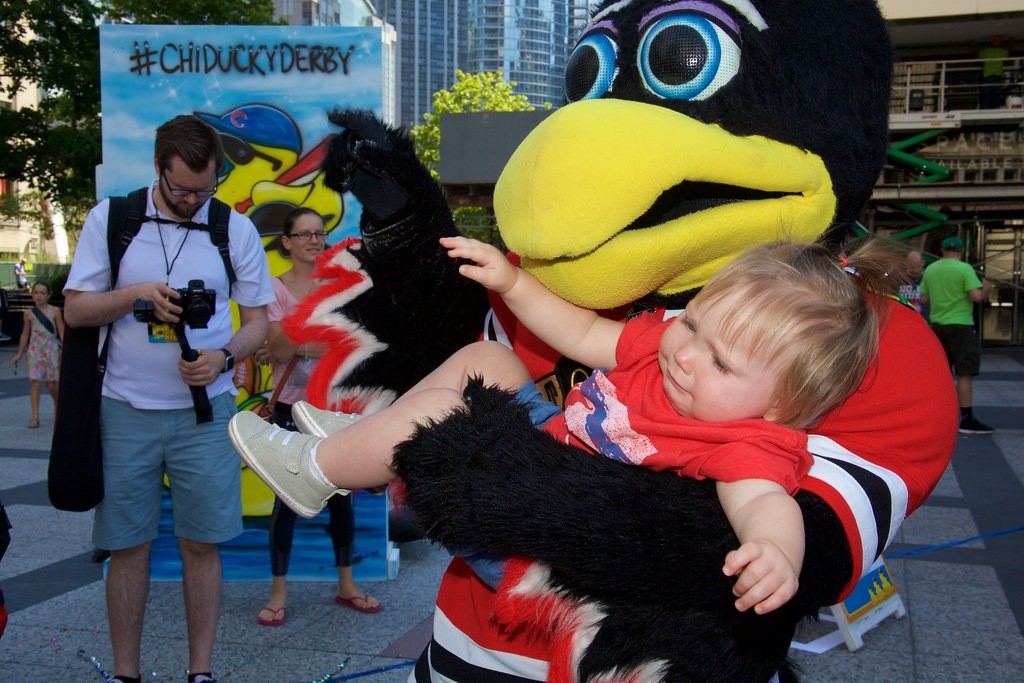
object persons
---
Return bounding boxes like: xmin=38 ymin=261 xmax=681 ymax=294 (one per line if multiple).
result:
xmin=258 ymin=207 xmax=384 ymax=625
xmin=63 ymin=116 xmax=276 ymax=683
xmin=228 ymin=236 xmax=876 ymax=618
xmin=14 ymin=258 xmax=28 ymax=289
xmin=891 ymin=250 xmax=931 ymax=323
xmin=10 ymin=281 xmax=64 ymax=428
xmin=909 ymin=236 xmax=996 ymax=434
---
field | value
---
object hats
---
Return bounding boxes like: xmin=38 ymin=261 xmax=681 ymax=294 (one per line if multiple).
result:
xmin=941 ymin=237 xmax=963 ymax=252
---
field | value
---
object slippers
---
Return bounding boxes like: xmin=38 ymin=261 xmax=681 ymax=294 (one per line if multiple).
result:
xmin=257 ymin=606 xmax=287 ymax=625
xmin=335 ymin=593 xmax=383 ymax=614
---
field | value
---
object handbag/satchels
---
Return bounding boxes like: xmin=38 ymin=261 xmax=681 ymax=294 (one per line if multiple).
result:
xmin=47 ymin=275 xmax=126 ymax=511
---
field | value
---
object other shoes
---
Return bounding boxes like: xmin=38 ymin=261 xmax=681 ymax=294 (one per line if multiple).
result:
xmin=188 ymin=672 xmax=218 ymax=683
xmin=106 ymin=672 xmax=142 ymax=683
xmin=28 ymin=418 xmax=40 ymax=429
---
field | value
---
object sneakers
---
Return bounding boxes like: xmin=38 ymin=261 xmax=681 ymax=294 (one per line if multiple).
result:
xmin=229 ymin=400 xmax=389 ymax=519
xmin=959 ymin=414 xmax=995 ymax=435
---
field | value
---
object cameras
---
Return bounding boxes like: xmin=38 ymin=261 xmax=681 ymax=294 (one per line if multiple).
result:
xmin=169 ymin=280 xmax=216 ymax=330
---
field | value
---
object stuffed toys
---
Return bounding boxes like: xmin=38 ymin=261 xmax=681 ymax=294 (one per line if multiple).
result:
xmin=282 ymin=5 xmax=957 ymax=683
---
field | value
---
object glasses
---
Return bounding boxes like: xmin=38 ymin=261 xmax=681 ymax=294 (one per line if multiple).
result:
xmin=162 ymin=165 xmax=218 ymax=197
xmin=284 ymin=229 xmax=329 ymax=240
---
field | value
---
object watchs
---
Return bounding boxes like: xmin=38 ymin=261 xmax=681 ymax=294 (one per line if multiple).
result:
xmin=220 ymin=346 xmax=234 ymax=373
